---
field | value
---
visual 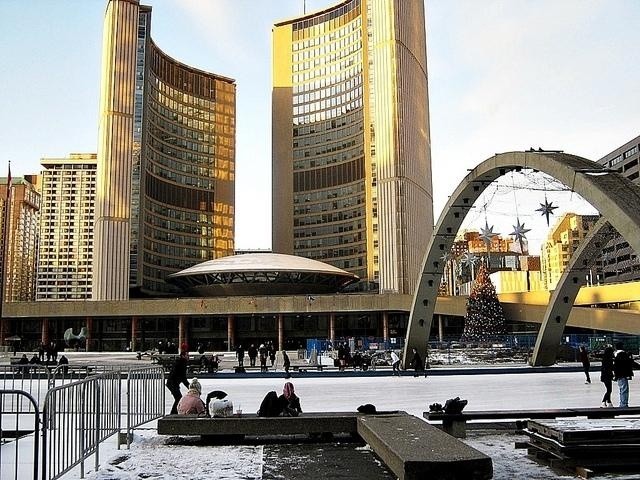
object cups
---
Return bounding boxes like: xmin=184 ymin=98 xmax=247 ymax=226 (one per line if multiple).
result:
xmin=236 ymin=410 xmax=242 ymax=417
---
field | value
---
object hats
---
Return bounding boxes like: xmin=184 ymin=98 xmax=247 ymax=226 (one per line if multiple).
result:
xmin=189 ymin=378 xmax=201 ymax=395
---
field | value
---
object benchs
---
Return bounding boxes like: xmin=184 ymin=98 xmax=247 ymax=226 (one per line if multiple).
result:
xmin=11 ymin=364 xmax=93 ymax=373
xmin=159 ymin=409 xmax=407 ymax=440
xmin=357 ymin=414 xmax=494 ymax=479
xmin=232 ymin=365 xmax=273 ymax=375
xmin=423 ymin=405 xmax=639 ymax=437
xmin=338 ymin=364 xmax=364 ymax=371
xmin=285 ymin=365 xmax=326 ymax=374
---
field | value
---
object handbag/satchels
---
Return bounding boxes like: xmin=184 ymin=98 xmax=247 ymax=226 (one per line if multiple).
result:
xmin=206 ymin=391 xmax=233 ymax=418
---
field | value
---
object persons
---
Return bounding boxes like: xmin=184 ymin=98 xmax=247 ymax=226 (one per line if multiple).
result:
xmin=601 ymin=348 xmax=614 ymax=407
xmin=12 ymin=340 xmax=69 ymax=378
xmin=612 ymin=342 xmax=634 ymax=408
xmin=278 ymin=382 xmax=303 ymax=413
xmin=158 ymin=339 xmax=428 ymax=377
xmin=176 ymin=377 xmax=205 ymax=415
xmin=580 ymin=347 xmax=591 ymax=384
xmin=165 ymin=351 xmax=190 ymax=414
xmin=273 ymin=400 xmax=300 ymax=417
